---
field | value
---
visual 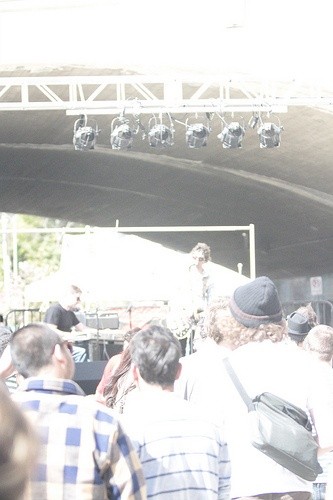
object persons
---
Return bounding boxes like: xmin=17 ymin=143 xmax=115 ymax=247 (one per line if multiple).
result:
xmin=0 ymin=276 xmax=333 ymax=500
xmin=186 ymin=243 xmax=211 ymax=315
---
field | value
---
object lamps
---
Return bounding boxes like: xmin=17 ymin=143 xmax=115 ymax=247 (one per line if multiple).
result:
xmin=73 ymin=118 xmax=101 ymax=150
xmin=217 ymin=116 xmax=246 ymax=149
xmin=258 ymin=111 xmax=282 ymax=150
xmin=146 ymin=117 xmax=174 ymax=149
xmin=184 ymin=119 xmax=211 ymax=149
xmin=110 ymin=117 xmax=138 ymax=151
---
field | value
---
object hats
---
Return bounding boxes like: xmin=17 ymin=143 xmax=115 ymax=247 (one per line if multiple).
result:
xmin=230 ymin=277 xmax=283 ymax=326
xmin=287 ymin=312 xmax=311 ymax=335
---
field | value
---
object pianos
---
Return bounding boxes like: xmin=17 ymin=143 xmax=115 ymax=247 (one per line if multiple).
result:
xmin=67 ymin=332 xmax=126 ymax=361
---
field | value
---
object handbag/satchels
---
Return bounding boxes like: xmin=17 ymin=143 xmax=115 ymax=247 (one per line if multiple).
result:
xmin=249 ymin=394 xmax=321 ymax=482
